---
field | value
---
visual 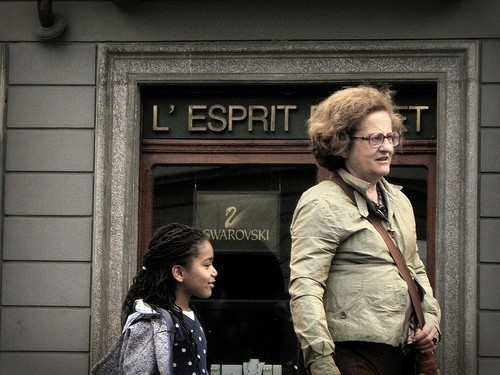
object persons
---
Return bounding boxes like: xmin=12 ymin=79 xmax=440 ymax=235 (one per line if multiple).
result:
xmin=290 ymin=86 xmax=441 ymax=375
xmin=90 ymin=223 xmax=218 ymax=375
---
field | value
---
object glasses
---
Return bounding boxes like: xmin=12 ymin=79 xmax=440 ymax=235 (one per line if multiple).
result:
xmin=352 ymin=131 xmax=401 ymax=148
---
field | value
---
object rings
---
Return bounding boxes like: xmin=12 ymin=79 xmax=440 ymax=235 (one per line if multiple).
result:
xmin=432 ymin=337 xmax=437 ymax=344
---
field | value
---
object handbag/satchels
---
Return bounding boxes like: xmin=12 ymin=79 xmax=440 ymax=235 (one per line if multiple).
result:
xmin=411 ymin=344 xmax=441 ymax=375
